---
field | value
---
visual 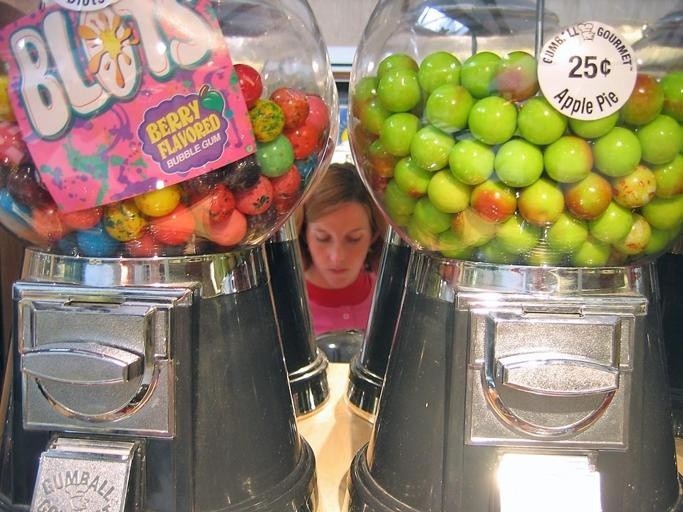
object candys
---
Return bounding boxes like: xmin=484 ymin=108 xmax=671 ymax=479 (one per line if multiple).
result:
xmin=0 ymin=65 xmax=334 ymax=257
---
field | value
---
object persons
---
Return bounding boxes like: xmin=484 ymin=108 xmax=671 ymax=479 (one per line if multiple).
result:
xmin=302 ymin=163 xmax=391 ymax=339
